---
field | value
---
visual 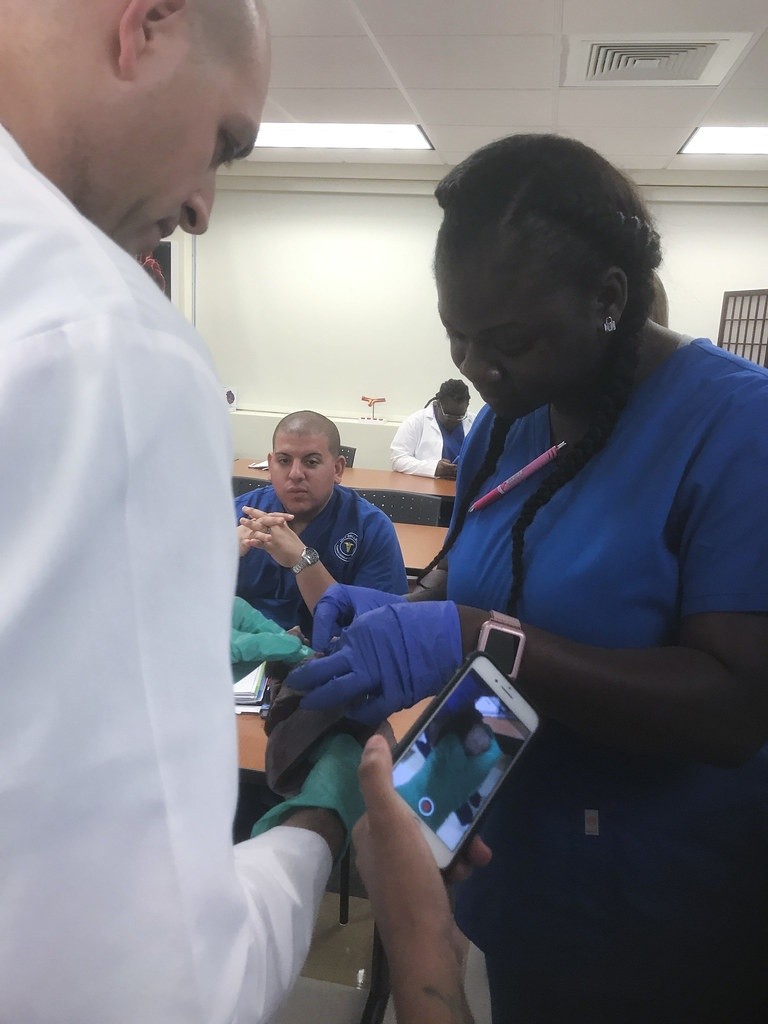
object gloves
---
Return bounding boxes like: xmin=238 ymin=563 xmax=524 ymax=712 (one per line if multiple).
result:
xmin=251 ymin=733 xmax=367 ymax=871
xmin=424 ymin=675 xmax=493 ymax=745
xmin=229 ymin=594 xmax=315 ymax=684
xmin=285 ymin=583 xmax=463 ymax=725
xmin=394 ymin=723 xmax=503 ymax=833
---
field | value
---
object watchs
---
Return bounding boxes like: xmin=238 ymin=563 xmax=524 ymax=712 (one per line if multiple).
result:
xmin=477 ymin=610 xmax=526 ymax=682
xmin=290 ymin=547 xmax=320 ymax=575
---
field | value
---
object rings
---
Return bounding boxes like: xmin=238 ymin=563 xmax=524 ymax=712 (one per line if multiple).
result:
xmin=266 ymin=526 xmax=271 ymax=534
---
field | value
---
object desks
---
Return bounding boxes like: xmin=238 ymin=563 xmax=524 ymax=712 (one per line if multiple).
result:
xmin=231 ymin=459 xmax=455 ymax=502
xmin=227 ymin=692 xmax=440 ymax=926
xmin=391 ymin=523 xmax=449 ymax=576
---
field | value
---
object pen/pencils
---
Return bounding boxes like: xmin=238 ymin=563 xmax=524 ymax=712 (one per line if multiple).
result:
xmin=466 ymin=441 xmax=568 ymax=515
xmin=339 ymin=846 xmax=350 ymax=927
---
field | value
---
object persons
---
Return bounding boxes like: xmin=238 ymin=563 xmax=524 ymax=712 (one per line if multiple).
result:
xmin=349 ymin=729 xmax=494 ymax=1024
xmin=393 ymin=734 xmax=501 ymax=832
xmin=235 ymin=408 xmax=408 ymax=645
xmin=389 ymin=378 xmax=477 ymax=481
xmin=282 ymin=131 xmax=768 ymax=1024
xmin=0 ymin=0 xmax=355 ymax=1024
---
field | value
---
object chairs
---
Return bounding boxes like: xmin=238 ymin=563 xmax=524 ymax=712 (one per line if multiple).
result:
xmin=339 ymin=446 xmax=358 ymax=468
xmin=232 ymin=476 xmax=274 ymax=497
xmin=351 ymin=488 xmax=443 ymax=527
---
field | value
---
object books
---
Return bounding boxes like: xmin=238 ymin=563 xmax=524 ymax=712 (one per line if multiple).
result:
xmin=231 ymin=659 xmax=270 ymax=705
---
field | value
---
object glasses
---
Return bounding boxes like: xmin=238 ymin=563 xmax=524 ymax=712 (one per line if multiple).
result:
xmin=440 ymin=401 xmax=467 ymax=421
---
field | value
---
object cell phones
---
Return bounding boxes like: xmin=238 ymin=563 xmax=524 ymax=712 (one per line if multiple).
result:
xmin=394 ymin=649 xmax=538 ymax=877
xmin=452 ymin=456 xmax=459 ymax=464
